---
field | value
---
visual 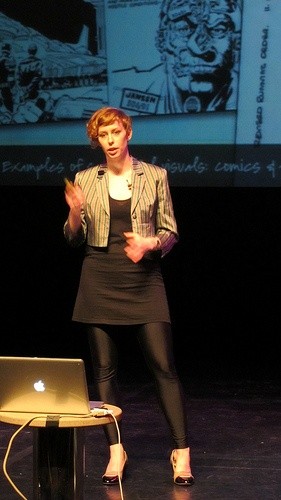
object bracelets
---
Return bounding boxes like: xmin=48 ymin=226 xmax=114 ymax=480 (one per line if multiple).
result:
xmin=155 ymin=237 xmax=161 ymax=251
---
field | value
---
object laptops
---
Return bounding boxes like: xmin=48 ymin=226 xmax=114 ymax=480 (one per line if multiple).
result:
xmin=0 ymin=357 xmax=104 ymax=417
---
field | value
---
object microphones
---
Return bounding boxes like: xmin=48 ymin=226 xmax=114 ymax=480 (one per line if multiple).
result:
xmin=98 ymin=170 xmax=104 ymax=175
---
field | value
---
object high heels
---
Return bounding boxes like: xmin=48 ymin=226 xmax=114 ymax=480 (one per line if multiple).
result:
xmin=170 ymin=449 xmax=194 ymax=486
xmin=102 ymin=451 xmax=128 ymax=485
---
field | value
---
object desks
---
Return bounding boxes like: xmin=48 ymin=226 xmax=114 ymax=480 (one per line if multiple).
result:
xmin=0 ymin=402 xmax=122 ymax=500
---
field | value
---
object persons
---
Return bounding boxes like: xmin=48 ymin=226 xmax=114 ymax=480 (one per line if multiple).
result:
xmin=63 ymin=106 xmax=194 ymax=486
